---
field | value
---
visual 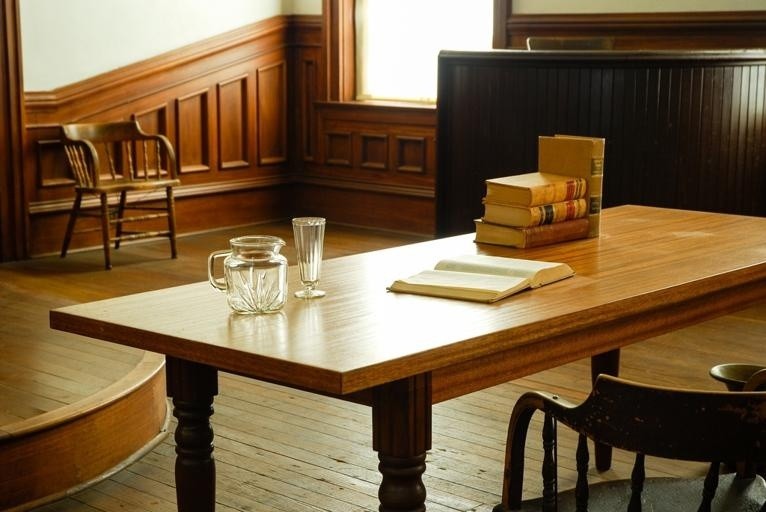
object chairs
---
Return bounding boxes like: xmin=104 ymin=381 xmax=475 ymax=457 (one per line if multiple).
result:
xmin=59 ymin=114 xmax=180 ymax=270
xmin=528 ymin=37 xmax=614 ymax=49
xmin=491 ymin=369 xmax=766 ymax=512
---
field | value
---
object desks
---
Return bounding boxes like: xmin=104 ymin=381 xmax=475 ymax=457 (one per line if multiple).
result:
xmin=50 ymin=205 xmax=766 ymax=512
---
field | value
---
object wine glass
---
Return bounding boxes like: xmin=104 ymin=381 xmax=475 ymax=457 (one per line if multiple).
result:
xmin=291 ymin=215 xmax=326 ymax=298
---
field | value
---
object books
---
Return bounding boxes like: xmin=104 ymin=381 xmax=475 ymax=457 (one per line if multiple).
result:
xmin=473 ymin=134 xmax=606 ymax=249
xmin=386 ymin=254 xmax=575 ymax=304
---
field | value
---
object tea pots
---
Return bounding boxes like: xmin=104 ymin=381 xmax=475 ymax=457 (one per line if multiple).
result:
xmin=206 ymin=234 xmax=289 ymax=314
